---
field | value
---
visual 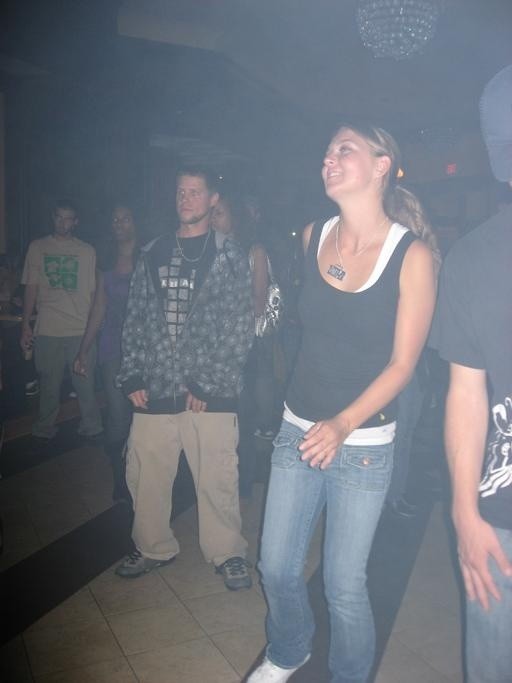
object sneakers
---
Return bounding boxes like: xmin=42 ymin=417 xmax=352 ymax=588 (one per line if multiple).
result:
xmin=254 ymin=428 xmax=275 ymax=440
xmin=112 ymin=472 xmax=129 ymax=504
xmin=246 ymin=647 xmax=312 ymax=683
xmin=24 ymin=379 xmax=105 ymax=448
xmin=114 ymin=549 xmax=176 ymax=579
xmin=215 ymin=553 xmax=253 ymax=592
xmin=391 ymin=493 xmax=417 ymax=519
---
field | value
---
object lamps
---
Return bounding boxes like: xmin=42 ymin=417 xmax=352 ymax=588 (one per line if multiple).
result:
xmin=356 ymin=0 xmax=442 ymax=62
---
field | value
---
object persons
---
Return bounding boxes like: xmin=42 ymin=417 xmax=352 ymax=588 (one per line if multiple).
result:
xmin=71 ymin=198 xmax=145 ymax=463
xmin=212 ymin=188 xmax=271 ymax=318
xmin=427 ymin=60 xmax=512 ymax=683
xmin=245 ymin=120 xmax=443 ymax=683
xmin=20 ymin=195 xmax=111 ymax=451
xmin=114 ymin=161 xmax=258 ymax=594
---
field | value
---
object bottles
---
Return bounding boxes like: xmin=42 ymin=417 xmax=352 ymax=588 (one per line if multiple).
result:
xmin=23 ymin=350 xmax=32 ymax=361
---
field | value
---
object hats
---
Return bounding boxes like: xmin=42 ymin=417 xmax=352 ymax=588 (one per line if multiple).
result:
xmin=478 ymin=62 xmax=511 ymax=183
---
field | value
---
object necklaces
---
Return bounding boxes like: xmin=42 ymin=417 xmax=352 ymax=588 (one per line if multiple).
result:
xmin=175 ymin=226 xmax=211 ymax=263
xmin=326 ymin=213 xmax=391 ymax=280
